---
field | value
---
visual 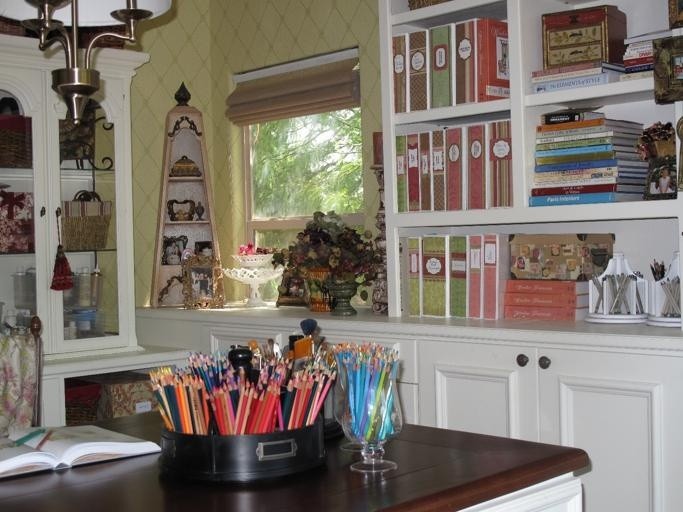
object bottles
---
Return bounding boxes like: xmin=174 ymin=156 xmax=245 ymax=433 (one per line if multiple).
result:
xmin=67 ymin=322 xmax=78 ymax=339
xmin=20 ymin=308 xmax=33 ymax=328
xmin=77 ymin=267 xmax=92 ymax=308
xmin=12 ymin=264 xmax=27 ymax=309
xmin=3 ymin=310 xmax=19 ymax=328
xmin=90 ymin=262 xmax=104 ymax=308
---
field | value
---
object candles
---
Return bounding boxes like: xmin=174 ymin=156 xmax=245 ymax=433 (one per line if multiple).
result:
xmin=370 ymin=129 xmax=382 ymax=167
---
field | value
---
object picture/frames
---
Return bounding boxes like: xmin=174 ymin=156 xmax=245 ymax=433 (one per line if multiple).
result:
xmin=650 ymin=34 xmax=682 ymax=105
xmin=177 ymin=253 xmax=225 ymax=310
xmin=159 ymin=234 xmax=189 ymax=266
xmin=275 ymin=265 xmax=305 ymax=306
xmin=644 ymin=153 xmax=679 ymax=202
xmin=666 ymin=1 xmax=682 ymax=30
xmin=193 ymin=239 xmax=211 ymax=258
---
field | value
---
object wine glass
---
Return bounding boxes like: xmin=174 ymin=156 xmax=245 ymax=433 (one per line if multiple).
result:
xmin=331 ymin=362 xmax=404 ymax=475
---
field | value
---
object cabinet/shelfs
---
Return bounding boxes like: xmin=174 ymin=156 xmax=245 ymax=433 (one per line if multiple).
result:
xmin=0 ymin=29 xmax=151 ymax=360
xmin=208 ymin=322 xmax=416 ymax=427
xmin=378 ymin=0 xmax=682 ymax=324
xmin=417 ymin=333 xmax=682 ymax=510
xmin=152 ymin=76 xmax=229 ymax=311
xmin=38 ymin=342 xmax=197 ymax=428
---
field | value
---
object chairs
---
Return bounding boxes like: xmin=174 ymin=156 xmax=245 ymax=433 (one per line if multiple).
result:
xmin=0 ymin=313 xmax=46 ymax=434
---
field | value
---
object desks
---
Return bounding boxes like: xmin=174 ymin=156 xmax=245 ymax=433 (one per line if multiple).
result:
xmin=0 ymin=401 xmax=592 ymax=510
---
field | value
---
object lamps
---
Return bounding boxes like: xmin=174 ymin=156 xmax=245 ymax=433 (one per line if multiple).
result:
xmin=1 ymin=0 xmax=184 ymax=135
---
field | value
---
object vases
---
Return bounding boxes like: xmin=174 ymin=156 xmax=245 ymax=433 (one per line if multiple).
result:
xmin=300 ymin=266 xmax=337 ymax=314
xmin=323 ymin=278 xmax=361 ymax=318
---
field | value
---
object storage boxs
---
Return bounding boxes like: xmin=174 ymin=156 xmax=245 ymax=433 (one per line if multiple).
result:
xmin=64 ymin=311 xmax=106 ymax=340
xmin=505 ymin=228 xmax=615 ymax=281
xmin=62 ymin=271 xmax=103 ymax=314
xmin=537 ymin=5 xmax=629 ymax=68
xmin=8 ymin=268 xmax=36 ymax=310
xmin=97 ymin=369 xmax=168 ymax=422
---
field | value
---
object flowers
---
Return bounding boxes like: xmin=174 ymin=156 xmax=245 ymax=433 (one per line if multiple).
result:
xmin=269 ymin=205 xmax=382 ymax=307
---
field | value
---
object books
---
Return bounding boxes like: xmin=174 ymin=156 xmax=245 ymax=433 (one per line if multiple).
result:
xmin=504 ymin=277 xmax=589 ymax=319
xmin=392 ymin=18 xmax=508 ymax=113
xmin=0 ymin=424 xmax=161 ymax=479
xmin=528 ymin=105 xmax=649 ymax=205
xmin=396 ymin=120 xmax=513 ymax=213
xmin=400 ymin=235 xmax=496 ymax=319
xmin=531 ymin=29 xmax=682 ymax=89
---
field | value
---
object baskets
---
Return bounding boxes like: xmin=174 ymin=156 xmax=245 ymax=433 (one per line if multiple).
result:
xmin=0 ymin=115 xmax=33 ymax=168
xmin=66 ymin=381 xmax=100 ymax=426
xmin=61 ymin=191 xmax=112 ymax=251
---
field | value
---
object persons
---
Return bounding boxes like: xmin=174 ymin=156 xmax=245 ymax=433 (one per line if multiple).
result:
xmin=195 ymin=202 xmax=203 ymax=214
xmin=166 ymin=241 xmax=208 ymax=295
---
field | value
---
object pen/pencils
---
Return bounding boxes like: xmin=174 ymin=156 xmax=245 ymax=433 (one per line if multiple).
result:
xmin=651 ymin=259 xmax=681 ymax=318
xmin=591 ymin=271 xmax=645 ymax=317
xmin=148 ymin=317 xmax=335 ymax=435
xmin=334 ymin=343 xmax=404 ymax=445
